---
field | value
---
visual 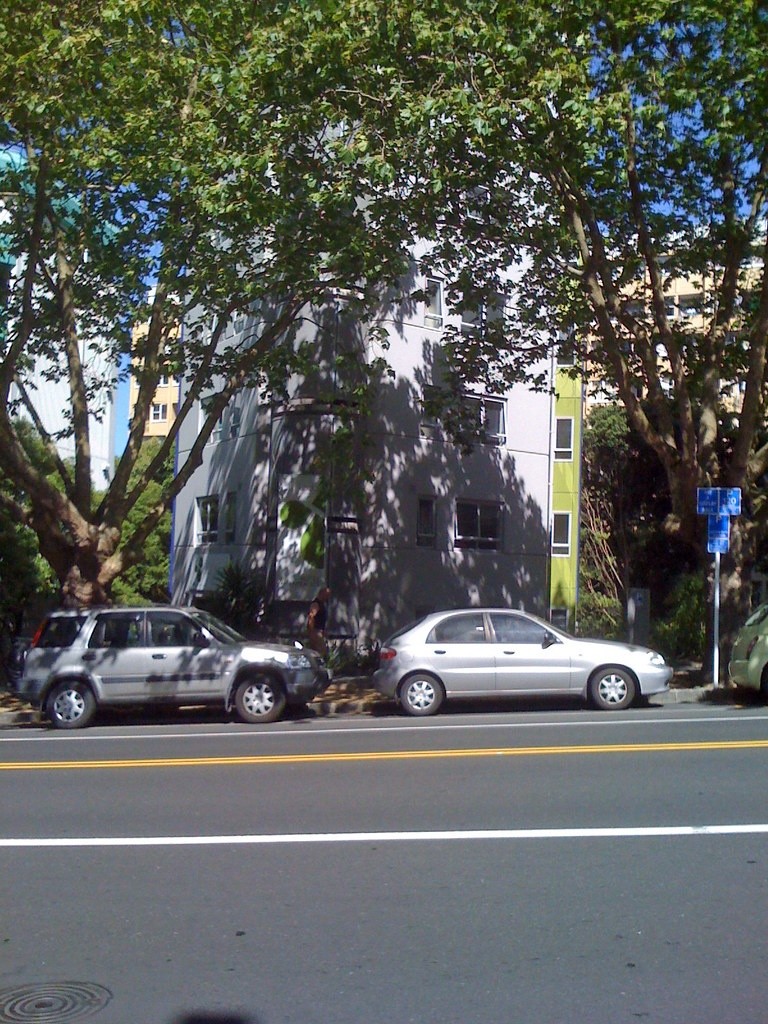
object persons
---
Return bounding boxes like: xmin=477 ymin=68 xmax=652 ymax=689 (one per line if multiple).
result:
xmin=307 ymin=587 xmax=332 ymax=658
xmin=4 ymin=594 xmax=27 ymax=686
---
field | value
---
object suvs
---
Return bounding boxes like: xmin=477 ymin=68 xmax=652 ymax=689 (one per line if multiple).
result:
xmin=11 ymin=605 xmax=336 ymax=729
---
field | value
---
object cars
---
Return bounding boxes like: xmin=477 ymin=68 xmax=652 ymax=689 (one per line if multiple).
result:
xmin=729 ymin=602 xmax=768 ymax=700
xmin=374 ymin=608 xmax=672 ymax=716
